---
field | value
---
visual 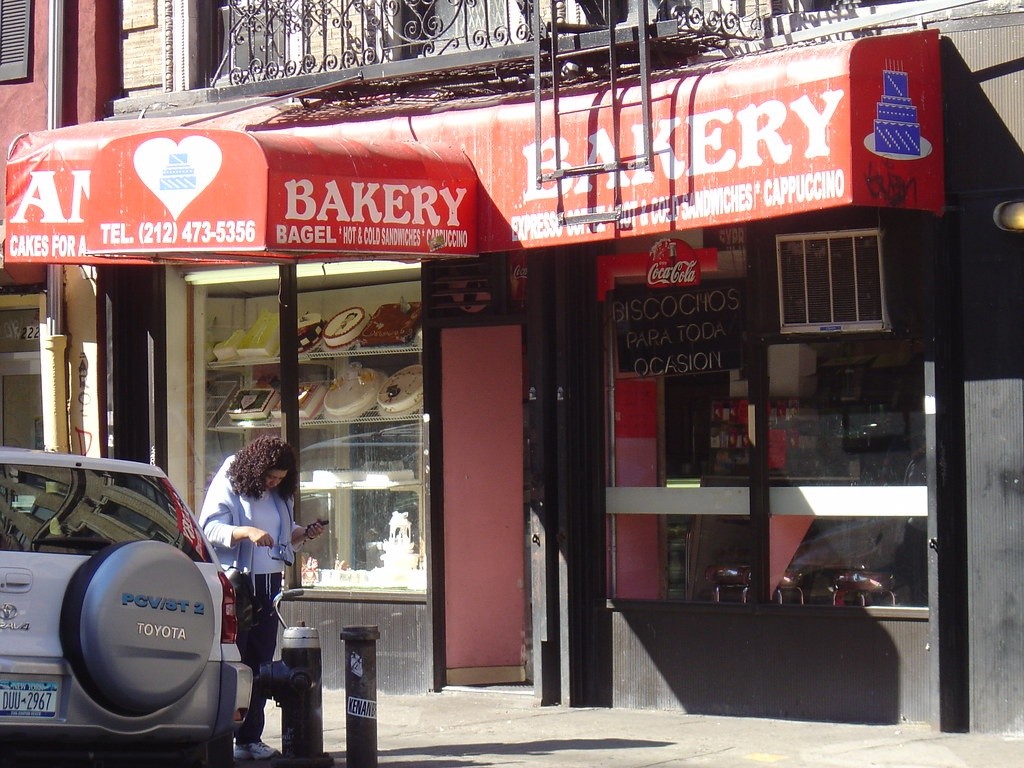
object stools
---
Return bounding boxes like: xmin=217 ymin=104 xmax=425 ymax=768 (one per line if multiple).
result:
xmin=707 ymin=567 xmax=897 ymax=606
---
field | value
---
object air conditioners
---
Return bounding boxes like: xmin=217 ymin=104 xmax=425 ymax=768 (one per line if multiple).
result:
xmin=773 ymin=227 xmax=894 ymax=334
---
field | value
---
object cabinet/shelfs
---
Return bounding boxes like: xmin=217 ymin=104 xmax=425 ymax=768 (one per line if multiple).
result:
xmin=205 ymin=345 xmax=422 ymax=591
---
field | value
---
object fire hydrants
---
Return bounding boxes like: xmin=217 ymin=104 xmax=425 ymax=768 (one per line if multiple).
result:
xmin=252 ymin=627 xmax=336 ymax=768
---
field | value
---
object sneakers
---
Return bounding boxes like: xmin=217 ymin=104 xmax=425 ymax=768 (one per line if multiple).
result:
xmin=234 ymin=738 xmax=277 ymax=759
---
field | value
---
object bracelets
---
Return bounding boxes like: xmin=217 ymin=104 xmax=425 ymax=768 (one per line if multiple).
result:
xmin=304 ymin=527 xmax=314 ymax=541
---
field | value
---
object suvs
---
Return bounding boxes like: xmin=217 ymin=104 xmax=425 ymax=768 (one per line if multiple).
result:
xmin=299 ymin=423 xmax=424 ymax=557
xmin=1 ymin=445 xmax=240 ymax=768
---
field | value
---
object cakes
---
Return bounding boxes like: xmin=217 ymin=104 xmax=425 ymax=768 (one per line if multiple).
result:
xmin=213 ymin=328 xmax=246 ymax=359
xmin=323 ymin=361 xmax=382 ymax=416
xmin=322 ymin=305 xmax=372 ymax=348
xmin=236 ymin=308 xmax=278 ymax=356
xmin=299 ymin=467 xmax=424 ymax=588
xmin=270 ymin=381 xmax=327 ymax=419
xmin=226 ymin=388 xmax=276 ymax=423
xmin=377 ymin=364 xmax=423 ymax=415
xmin=357 ymin=295 xmax=422 ymax=345
xmin=297 ymin=312 xmax=327 ymax=353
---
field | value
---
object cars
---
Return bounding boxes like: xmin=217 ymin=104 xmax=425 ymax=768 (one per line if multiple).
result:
xmin=314 ymin=496 xmax=426 ymax=573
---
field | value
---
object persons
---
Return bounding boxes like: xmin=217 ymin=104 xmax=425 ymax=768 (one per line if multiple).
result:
xmin=199 ymin=435 xmax=325 ymax=760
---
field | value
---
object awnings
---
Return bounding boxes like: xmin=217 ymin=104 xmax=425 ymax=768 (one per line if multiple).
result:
xmin=5 ymin=26 xmax=944 ymax=266
